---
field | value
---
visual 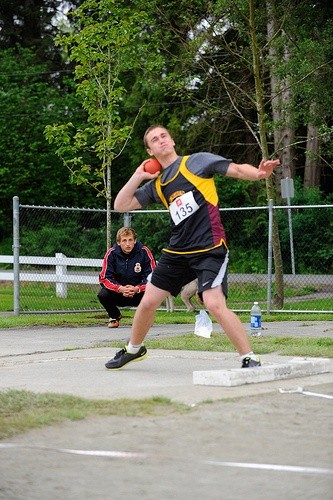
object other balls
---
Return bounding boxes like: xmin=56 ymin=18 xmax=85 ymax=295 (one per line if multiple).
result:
xmin=144 ymin=158 xmax=161 ymax=174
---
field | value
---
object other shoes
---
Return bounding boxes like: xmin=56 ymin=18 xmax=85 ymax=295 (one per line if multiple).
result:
xmin=108 ymin=313 xmax=123 ymax=327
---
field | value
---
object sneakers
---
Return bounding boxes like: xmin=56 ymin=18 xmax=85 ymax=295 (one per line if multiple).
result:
xmin=104 ymin=346 xmax=147 ymax=369
xmin=241 ymin=355 xmax=261 ymax=369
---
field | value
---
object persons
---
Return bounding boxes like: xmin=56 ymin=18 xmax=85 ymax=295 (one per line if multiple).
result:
xmin=104 ymin=123 xmax=280 ymax=371
xmin=97 ymin=227 xmax=156 ymax=328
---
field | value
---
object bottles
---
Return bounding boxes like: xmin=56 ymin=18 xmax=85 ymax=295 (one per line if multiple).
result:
xmin=249 ymin=303 xmax=262 ymax=337
